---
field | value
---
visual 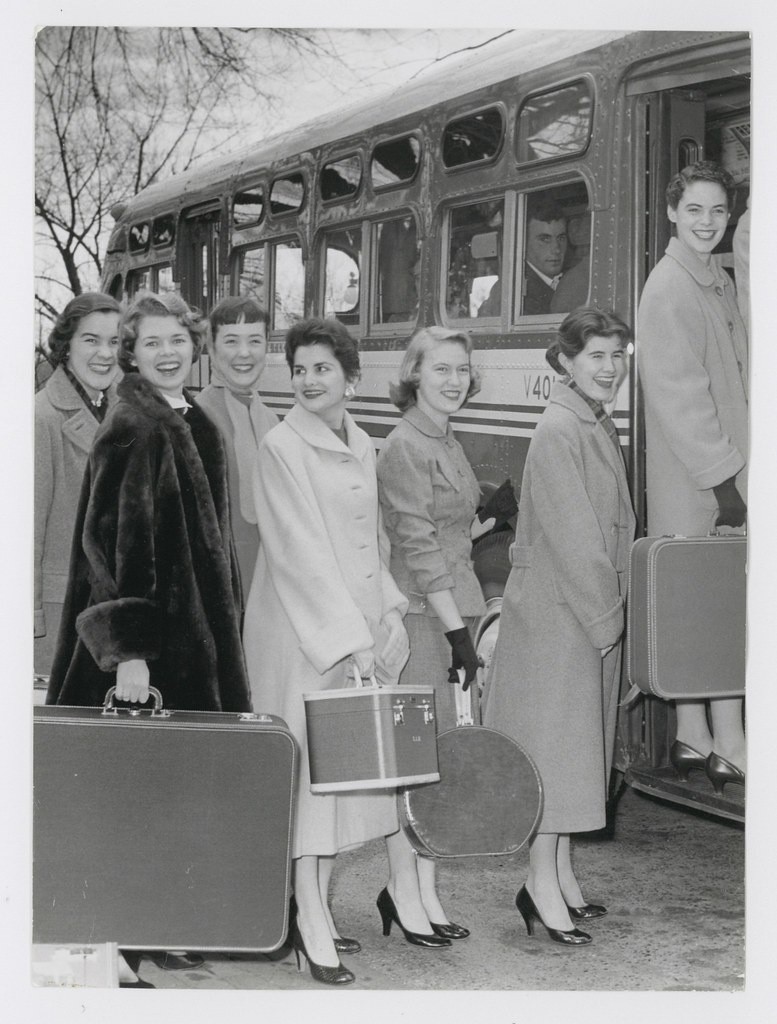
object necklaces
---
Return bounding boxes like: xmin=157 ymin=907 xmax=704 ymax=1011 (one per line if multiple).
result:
xmin=181 ymin=395 xmax=188 ymax=415
xmin=90 ymin=391 xmax=103 ymax=408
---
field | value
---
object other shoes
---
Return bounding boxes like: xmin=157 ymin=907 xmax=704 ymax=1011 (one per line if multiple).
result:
xmin=289 ymin=894 xmax=361 ymax=954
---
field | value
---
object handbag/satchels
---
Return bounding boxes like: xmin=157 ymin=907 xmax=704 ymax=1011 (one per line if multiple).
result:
xmin=395 ymin=669 xmax=543 ymax=857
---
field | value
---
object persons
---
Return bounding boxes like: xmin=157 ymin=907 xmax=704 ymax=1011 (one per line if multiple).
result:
xmin=638 ymin=161 xmax=747 ymax=798
xmin=375 ymin=325 xmax=487 ymax=950
xmin=241 ymin=319 xmax=410 ymax=984
xmin=47 ymin=292 xmax=255 ymax=988
xmin=477 ymin=205 xmax=568 ymax=318
xmin=483 ymin=304 xmax=635 ymax=947
xmin=195 ymin=298 xmax=281 ymax=582
xmin=732 ymin=194 xmax=751 ymax=332
xmin=550 ymin=201 xmax=592 ymax=314
xmin=34 ymin=294 xmax=125 ymax=684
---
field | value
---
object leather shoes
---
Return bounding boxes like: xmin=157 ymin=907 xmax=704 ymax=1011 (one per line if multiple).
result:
xmin=119 ymin=977 xmax=155 ymax=988
xmin=147 ymin=950 xmax=205 ymax=971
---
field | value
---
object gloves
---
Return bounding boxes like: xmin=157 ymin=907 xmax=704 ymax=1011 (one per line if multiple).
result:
xmin=478 ymin=478 xmax=520 ymax=533
xmin=712 ymin=476 xmax=747 ymax=529
xmin=444 ymin=626 xmax=479 ymax=691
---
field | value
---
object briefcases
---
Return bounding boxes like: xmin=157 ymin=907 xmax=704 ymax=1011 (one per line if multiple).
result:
xmin=628 ymin=514 xmax=746 ymax=699
xmin=31 ymin=684 xmax=301 ymax=952
xmin=302 ymin=664 xmax=439 ymax=794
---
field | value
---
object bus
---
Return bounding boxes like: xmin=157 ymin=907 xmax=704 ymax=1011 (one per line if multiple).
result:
xmin=98 ymin=29 xmax=749 ymax=831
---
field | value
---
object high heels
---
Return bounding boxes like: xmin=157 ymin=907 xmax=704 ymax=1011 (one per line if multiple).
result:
xmin=516 ymin=883 xmax=593 ymax=946
xmin=429 ymin=920 xmax=470 ymax=939
xmin=669 ymin=739 xmax=708 ymax=783
xmin=705 ymin=751 xmax=745 ymax=795
xmin=290 ymin=916 xmax=356 ymax=986
xmin=566 ymin=903 xmax=608 ymax=918
xmin=377 ymin=886 xmax=453 ymax=948
